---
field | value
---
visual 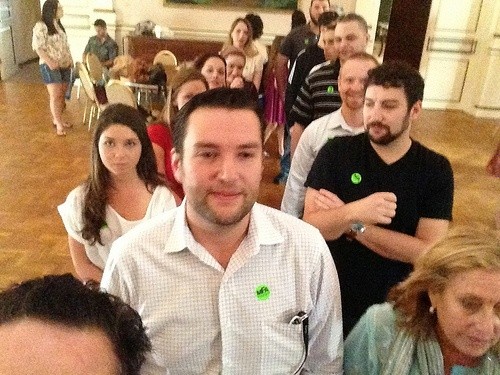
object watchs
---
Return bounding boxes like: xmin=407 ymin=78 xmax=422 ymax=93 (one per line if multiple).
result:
xmin=346 ymin=223 xmax=365 ymax=241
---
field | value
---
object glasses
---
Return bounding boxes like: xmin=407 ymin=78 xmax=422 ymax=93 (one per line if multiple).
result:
xmin=289 ymin=311 xmax=309 ymax=375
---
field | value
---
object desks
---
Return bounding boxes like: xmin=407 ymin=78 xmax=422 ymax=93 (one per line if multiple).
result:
xmin=119 ymin=77 xmax=174 ymax=132
xmin=127 ymin=35 xmax=272 ymax=65
xmin=378 ymin=21 xmax=389 ymax=57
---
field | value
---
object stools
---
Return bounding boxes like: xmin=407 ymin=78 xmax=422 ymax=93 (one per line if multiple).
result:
xmin=74 ymin=78 xmax=95 ymax=103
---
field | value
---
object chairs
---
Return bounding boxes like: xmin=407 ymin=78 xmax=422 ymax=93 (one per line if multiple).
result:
xmin=152 ymin=49 xmax=177 ymax=67
xmin=104 ymin=79 xmax=137 ymax=109
xmin=76 ymin=52 xmax=105 ymax=131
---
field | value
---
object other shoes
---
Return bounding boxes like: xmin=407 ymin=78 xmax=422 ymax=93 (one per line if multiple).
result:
xmin=273 ymin=170 xmax=288 ymax=184
xmin=278 ymin=148 xmax=285 ymax=158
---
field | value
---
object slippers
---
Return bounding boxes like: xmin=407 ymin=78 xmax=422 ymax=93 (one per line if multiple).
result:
xmin=57 ymin=125 xmax=66 ymax=135
xmin=53 ymin=121 xmax=73 ymax=127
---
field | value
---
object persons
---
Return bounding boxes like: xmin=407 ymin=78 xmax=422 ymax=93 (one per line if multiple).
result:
xmin=303 ymin=61 xmax=453 ymax=342
xmin=31 ymin=0 xmax=73 ymax=136
xmin=0 ymin=273 xmax=153 ymax=375
xmin=58 ymin=104 xmax=182 ymax=285
xmin=83 ymin=4 xmax=379 ymax=219
xmin=342 ymin=221 xmax=500 ymax=375
xmin=98 ymin=85 xmax=341 ymax=375
